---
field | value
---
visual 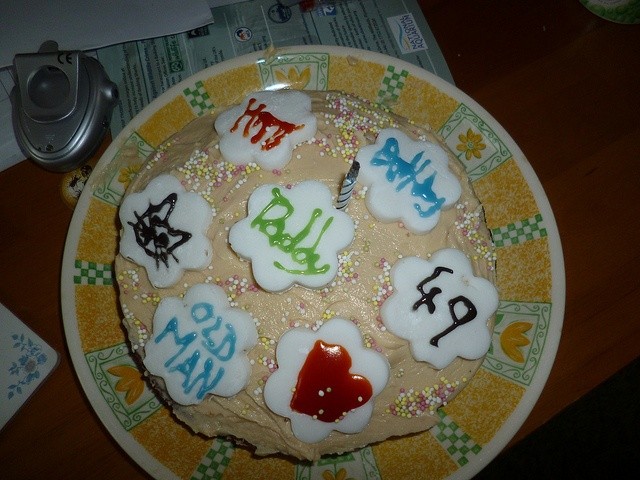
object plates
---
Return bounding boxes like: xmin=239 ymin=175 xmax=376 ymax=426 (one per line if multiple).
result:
xmin=59 ymin=45 xmax=568 ymax=480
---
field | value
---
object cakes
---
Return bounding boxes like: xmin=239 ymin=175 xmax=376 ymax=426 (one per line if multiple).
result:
xmin=113 ymin=86 xmax=500 ymax=462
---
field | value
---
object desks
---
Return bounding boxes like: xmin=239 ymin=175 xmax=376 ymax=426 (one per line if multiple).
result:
xmin=1 ymin=1 xmax=640 ymax=479
